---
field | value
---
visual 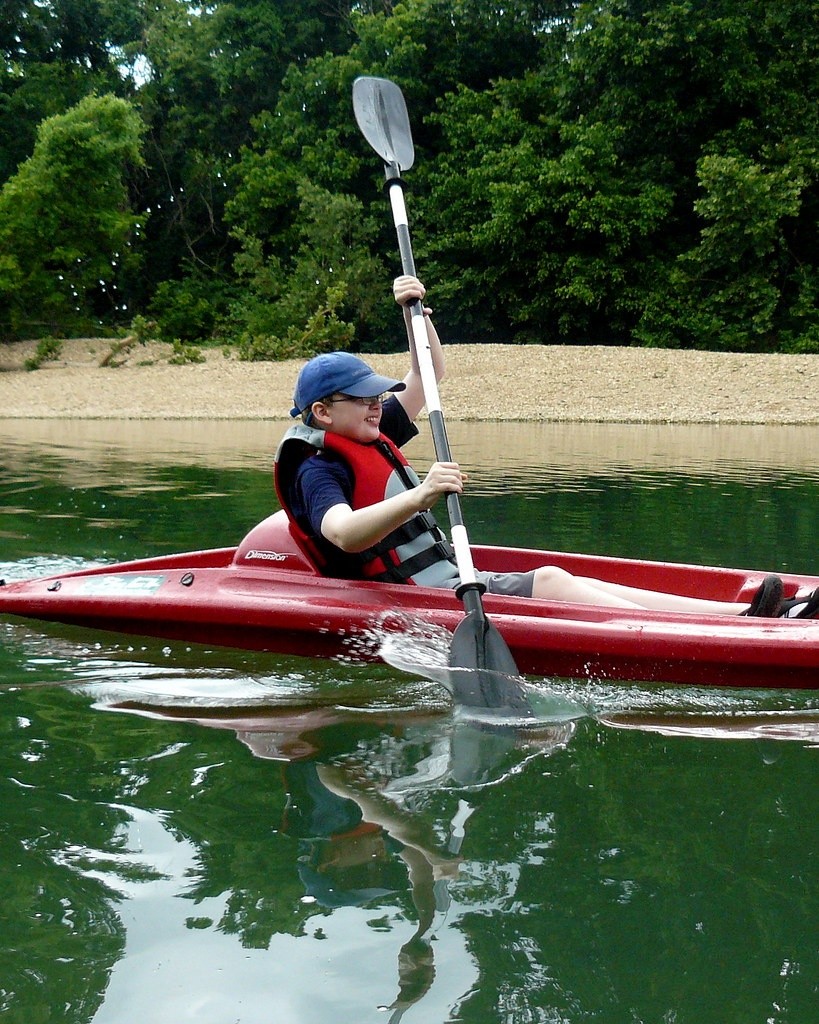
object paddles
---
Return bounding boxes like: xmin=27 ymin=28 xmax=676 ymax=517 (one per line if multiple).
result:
xmin=349 ymin=75 xmax=533 ymax=707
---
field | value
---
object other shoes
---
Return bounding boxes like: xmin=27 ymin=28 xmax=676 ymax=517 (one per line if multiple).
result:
xmin=738 ymin=576 xmax=783 ymax=617
xmin=778 ymin=586 xmax=818 ymax=618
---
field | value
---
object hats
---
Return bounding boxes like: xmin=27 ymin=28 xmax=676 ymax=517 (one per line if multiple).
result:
xmin=290 ymin=352 xmax=407 ymax=418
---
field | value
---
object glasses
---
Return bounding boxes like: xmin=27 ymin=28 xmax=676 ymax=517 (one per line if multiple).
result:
xmin=325 ymin=395 xmax=384 ymax=405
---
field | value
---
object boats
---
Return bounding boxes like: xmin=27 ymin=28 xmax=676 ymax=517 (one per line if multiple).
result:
xmin=0 ymin=509 xmax=819 ymax=690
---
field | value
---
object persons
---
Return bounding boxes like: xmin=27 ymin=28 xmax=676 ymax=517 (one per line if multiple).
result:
xmin=281 ymin=751 xmax=474 ymax=1000
xmin=274 ymin=275 xmax=819 ymax=619
